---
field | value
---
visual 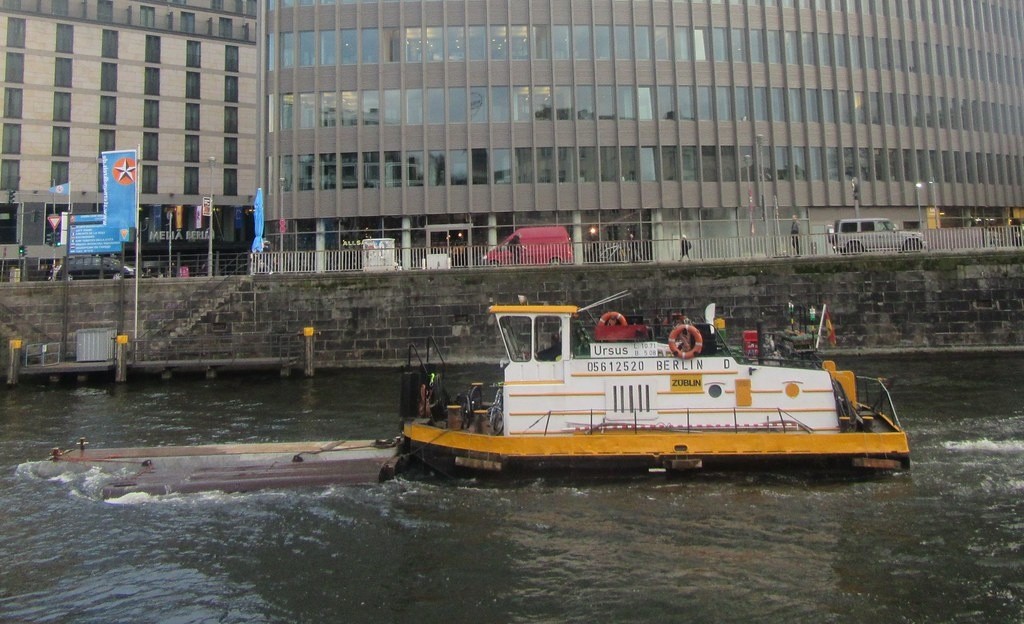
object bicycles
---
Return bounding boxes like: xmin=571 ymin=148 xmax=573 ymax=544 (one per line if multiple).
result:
xmin=458 ymin=381 xmax=504 ymax=435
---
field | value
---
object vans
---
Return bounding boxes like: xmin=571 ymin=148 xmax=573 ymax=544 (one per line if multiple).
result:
xmin=68 ymin=257 xmax=136 ymax=281
xmin=484 ymin=226 xmax=574 ymax=266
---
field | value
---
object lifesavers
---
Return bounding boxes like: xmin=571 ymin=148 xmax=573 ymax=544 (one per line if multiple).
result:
xmin=597 ymin=312 xmax=627 ymax=326
xmin=668 ymin=324 xmax=703 ymax=359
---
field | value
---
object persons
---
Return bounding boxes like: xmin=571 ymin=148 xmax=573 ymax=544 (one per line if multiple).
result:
xmin=790 ymin=215 xmax=804 ymax=258
xmin=570 ymin=312 xmax=594 ymax=359
xmin=534 ymin=333 xmax=562 ymax=361
xmin=678 ymin=238 xmax=691 ymax=262
xmin=451 ymin=232 xmax=468 ymax=266
xmin=1010 ymin=212 xmax=1022 ymax=247
xmin=507 ymin=232 xmax=522 ymax=264
xmin=654 ymin=314 xmax=661 ymax=337
xmin=393 ymin=234 xmax=402 ymax=270
xmin=625 ymin=234 xmax=638 ymax=262
xmin=419 ymin=384 xmax=445 ymax=422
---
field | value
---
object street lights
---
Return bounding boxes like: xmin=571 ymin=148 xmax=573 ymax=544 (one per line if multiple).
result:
xmin=206 ymin=156 xmax=217 ymax=276
xmin=916 ymin=182 xmax=922 ymax=230
xmin=927 ymin=177 xmax=938 ymax=229
xmin=278 ymin=177 xmax=286 ymax=253
xmin=744 ymin=155 xmax=755 ymax=258
xmin=755 ymin=134 xmax=770 ymax=258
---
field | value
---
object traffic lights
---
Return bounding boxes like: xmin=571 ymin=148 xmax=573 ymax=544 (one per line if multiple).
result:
xmin=8 ymin=189 xmax=15 ymax=205
xmin=19 ymin=245 xmax=27 ymax=257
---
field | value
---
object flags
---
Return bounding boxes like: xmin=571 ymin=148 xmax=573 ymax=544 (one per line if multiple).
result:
xmin=825 ymin=310 xmax=837 ymax=346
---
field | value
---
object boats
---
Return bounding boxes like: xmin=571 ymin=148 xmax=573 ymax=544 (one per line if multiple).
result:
xmin=399 ymin=303 xmax=912 ymax=496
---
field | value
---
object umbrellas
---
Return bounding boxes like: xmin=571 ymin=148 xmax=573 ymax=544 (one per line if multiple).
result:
xmin=252 ymin=188 xmax=265 ymax=271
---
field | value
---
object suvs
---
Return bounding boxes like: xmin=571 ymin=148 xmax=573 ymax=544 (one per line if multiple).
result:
xmin=826 ymin=217 xmax=926 ymax=255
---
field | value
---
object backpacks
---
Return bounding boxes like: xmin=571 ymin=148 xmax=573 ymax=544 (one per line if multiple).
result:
xmin=689 ymin=241 xmax=692 ymax=249
